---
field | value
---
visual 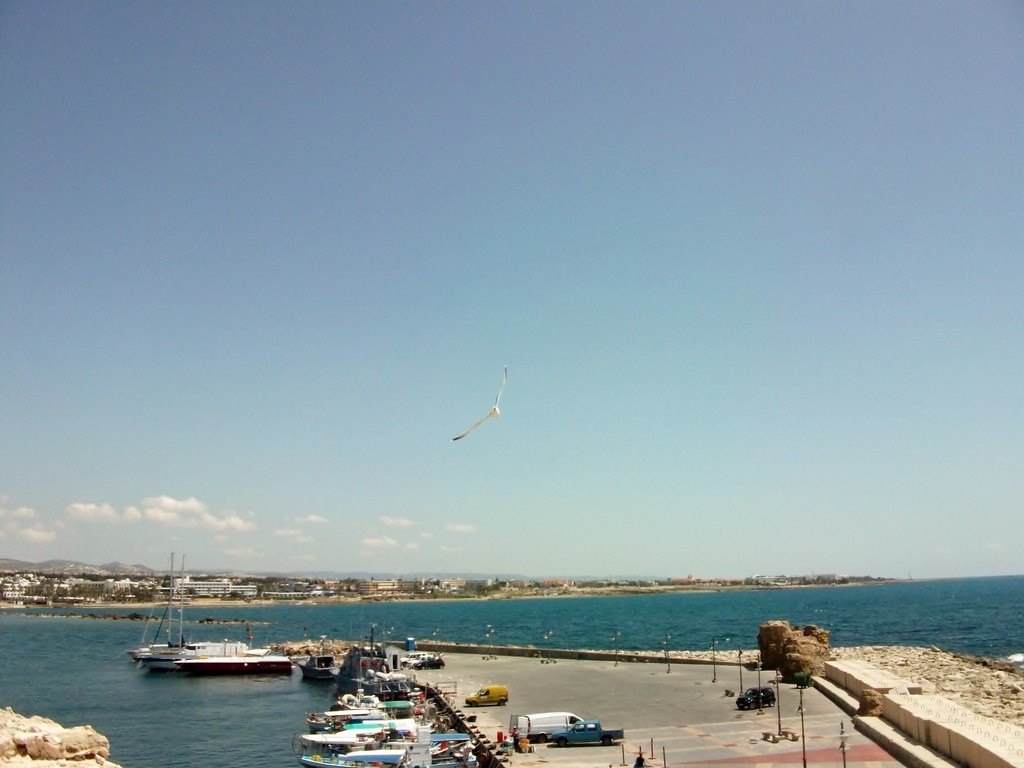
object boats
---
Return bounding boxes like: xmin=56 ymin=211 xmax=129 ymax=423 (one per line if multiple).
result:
xmin=126 ymin=644 xmax=292 ymax=673
xmin=294 ymin=695 xmax=480 ymax=768
xmin=296 ymin=643 xmax=415 ymax=694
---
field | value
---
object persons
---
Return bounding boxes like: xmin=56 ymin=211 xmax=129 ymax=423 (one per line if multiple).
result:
xmin=636 ymin=752 xmax=646 ymax=768
xmin=510 ymin=726 xmax=521 ymax=752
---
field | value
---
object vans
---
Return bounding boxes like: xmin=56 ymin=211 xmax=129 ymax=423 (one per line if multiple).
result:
xmin=464 ymin=686 xmax=508 ymax=708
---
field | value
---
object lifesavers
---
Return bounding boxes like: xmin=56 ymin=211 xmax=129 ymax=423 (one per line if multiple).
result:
xmin=398 ymin=729 xmax=410 ymax=735
xmin=420 ymin=693 xmax=425 ymax=701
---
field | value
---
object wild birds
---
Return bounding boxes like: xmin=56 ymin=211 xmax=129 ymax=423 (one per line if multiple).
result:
xmin=448 ymin=365 xmax=507 ymax=443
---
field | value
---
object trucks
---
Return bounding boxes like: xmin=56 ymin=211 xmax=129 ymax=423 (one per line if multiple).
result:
xmin=509 ymin=710 xmax=584 ymax=744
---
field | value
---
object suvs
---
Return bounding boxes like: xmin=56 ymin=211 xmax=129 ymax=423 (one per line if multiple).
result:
xmin=735 ymin=687 xmax=774 ymax=709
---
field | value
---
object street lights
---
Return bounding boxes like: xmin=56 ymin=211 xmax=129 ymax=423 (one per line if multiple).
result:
xmin=755 ymin=654 xmax=765 ymax=716
xmin=738 ymin=645 xmax=745 ymax=693
xmin=774 ymin=666 xmax=785 ymax=734
xmin=838 ymin=720 xmax=849 ymax=768
xmin=662 ymin=632 xmax=673 ymax=675
xmin=710 ymin=637 xmax=721 ymax=684
xmin=610 ymin=629 xmax=621 ymax=665
xmin=797 ymin=687 xmax=811 ymax=768
xmin=544 ymin=628 xmax=554 ymax=664
xmin=383 ymin=623 xmax=494 ymax=661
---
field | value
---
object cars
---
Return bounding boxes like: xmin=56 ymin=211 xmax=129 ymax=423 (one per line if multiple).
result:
xmin=399 ymin=652 xmax=445 ymax=669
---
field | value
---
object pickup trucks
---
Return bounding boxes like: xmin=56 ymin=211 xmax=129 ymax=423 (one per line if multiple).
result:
xmin=551 ymin=720 xmax=626 ymax=748
